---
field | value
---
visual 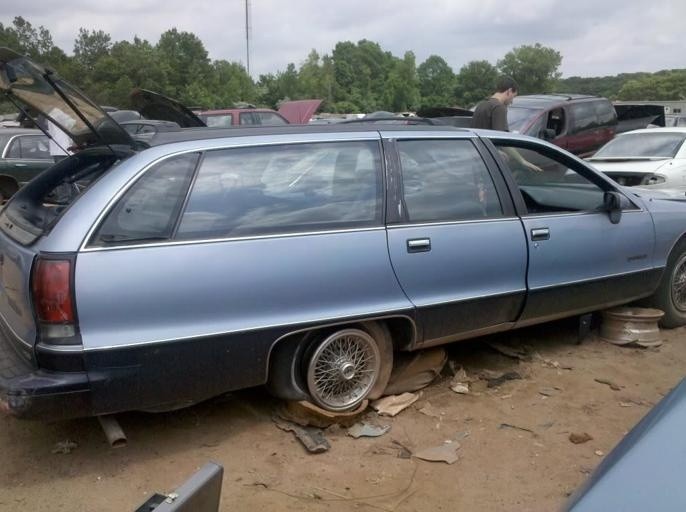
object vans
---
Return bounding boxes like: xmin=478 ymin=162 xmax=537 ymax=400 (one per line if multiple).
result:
xmin=0 ymin=46 xmax=686 ymax=425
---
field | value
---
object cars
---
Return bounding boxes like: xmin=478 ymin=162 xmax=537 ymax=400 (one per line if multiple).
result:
xmin=567 ymin=127 xmax=686 ymax=196
xmin=0 ymin=127 xmax=104 ymax=205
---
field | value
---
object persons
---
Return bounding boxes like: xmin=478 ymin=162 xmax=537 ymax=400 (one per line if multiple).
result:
xmin=46 ymin=98 xmax=87 ymax=205
xmin=470 ymin=74 xmax=545 ymax=174
